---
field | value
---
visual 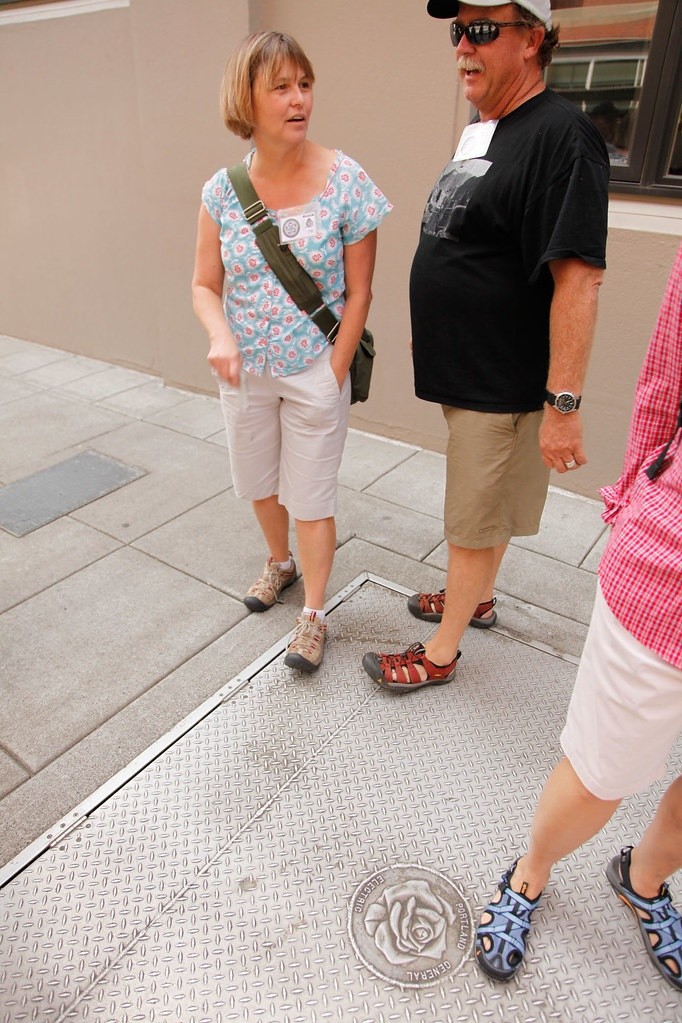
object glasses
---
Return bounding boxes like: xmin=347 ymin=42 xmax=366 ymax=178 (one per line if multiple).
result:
xmin=449 ymin=19 xmax=532 ymax=47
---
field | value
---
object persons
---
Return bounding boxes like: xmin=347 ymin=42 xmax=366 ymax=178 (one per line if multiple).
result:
xmin=474 ymin=244 xmax=682 ymax=993
xmin=192 ymin=30 xmax=393 ymax=670
xmin=364 ymin=0 xmax=610 ymax=693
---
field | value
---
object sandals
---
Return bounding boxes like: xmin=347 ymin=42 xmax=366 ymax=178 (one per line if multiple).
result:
xmin=408 ymin=590 xmax=498 ymax=628
xmin=605 ymin=845 xmax=682 ymax=991
xmin=362 ymin=641 xmax=462 ymax=693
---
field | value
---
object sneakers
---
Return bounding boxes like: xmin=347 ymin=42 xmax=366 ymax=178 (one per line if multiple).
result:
xmin=475 ymin=856 xmax=542 ymax=983
xmin=285 ymin=611 xmax=327 ymax=671
xmin=244 ymin=551 xmax=297 ymax=612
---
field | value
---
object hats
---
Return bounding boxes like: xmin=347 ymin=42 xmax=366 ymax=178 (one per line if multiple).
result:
xmin=427 ymin=0 xmax=553 ymax=31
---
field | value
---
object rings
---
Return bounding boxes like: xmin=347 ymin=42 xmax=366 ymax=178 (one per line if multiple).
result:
xmin=565 ymin=460 xmax=576 ymax=468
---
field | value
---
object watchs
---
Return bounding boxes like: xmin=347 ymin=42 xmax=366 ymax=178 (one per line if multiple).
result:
xmin=545 ymin=388 xmax=581 ymax=413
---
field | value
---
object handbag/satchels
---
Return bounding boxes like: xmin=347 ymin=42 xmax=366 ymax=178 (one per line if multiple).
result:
xmin=348 ymin=327 xmax=376 ymax=405
